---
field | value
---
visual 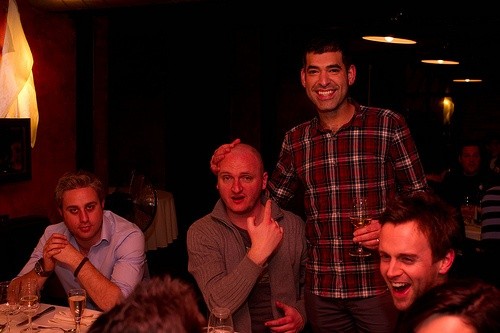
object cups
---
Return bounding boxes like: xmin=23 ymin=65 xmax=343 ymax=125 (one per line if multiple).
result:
xmin=208 ymin=308 xmax=233 ymax=333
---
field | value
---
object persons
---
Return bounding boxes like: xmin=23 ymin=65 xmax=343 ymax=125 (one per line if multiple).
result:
xmin=413 ymin=281 xmax=500 ymax=333
xmin=379 ymin=189 xmax=457 ymax=333
xmin=6 ymin=168 xmax=146 ymax=313
xmin=186 ymin=145 xmax=307 ymax=333
xmin=93 ymin=294 xmax=187 ymax=333
xmin=426 ymin=130 xmax=500 ymax=278
xmin=208 ymin=36 xmax=430 ymax=333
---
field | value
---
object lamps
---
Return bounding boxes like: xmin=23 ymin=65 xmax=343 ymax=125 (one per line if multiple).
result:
xmin=361 ymin=13 xmax=420 ymax=45
xmin=418 ymin=41 xmax=462 ymax=65
xmin=451 ymin=70 xmax=485 ymax=84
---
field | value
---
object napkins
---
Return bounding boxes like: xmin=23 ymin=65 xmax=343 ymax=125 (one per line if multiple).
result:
xmin=47 ymin=310 xmax=96 ymax=326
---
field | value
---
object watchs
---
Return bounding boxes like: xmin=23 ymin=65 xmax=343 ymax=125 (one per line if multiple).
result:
xmin=35 ymin=258 xmax=54 ymax=277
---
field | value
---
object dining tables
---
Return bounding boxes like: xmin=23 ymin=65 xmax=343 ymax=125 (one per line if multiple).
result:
xmin=0 ymin=303 xmax=104 ymax=333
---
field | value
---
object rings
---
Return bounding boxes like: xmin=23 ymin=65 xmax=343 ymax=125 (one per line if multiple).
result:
xmin=376 ymin=238 xmax=379 ymax=243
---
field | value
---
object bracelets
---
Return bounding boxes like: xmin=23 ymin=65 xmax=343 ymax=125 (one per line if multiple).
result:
xmin=73 ymin=256 xmax=89 ymax=278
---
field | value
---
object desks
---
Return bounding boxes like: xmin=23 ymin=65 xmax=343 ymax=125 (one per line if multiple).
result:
xmin=110 ymin=185 xmax=178 ymax=250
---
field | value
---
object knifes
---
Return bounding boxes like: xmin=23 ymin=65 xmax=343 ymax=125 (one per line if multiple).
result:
xmin=16 ymin=306 xmax=56 ymax=326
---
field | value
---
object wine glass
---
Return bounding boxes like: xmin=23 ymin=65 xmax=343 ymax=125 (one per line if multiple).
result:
xmin=16 ymin=278 xmax=40 ymax=333
xmin=67 ymin=289 xmax=86 ymax=333
xmin=0 ymin=281 xmax=19 ymax=333
xmin=348 ymin=196 xmax=372 ymax=257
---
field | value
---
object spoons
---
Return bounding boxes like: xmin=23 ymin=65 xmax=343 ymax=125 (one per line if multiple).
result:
xmin=38 ymin=326 xmax=76 ymax=333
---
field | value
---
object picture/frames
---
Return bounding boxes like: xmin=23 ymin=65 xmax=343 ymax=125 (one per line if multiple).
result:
xmin=0 ymin=118 xmax=33 ymax=186
